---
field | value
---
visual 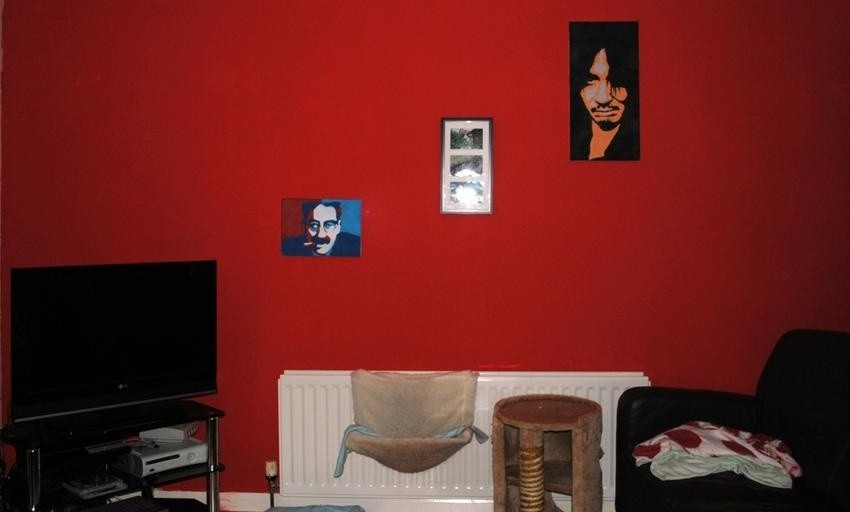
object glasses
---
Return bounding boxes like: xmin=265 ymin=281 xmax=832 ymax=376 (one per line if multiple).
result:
xmin=308 ymin=220 xmax=338 ymax=232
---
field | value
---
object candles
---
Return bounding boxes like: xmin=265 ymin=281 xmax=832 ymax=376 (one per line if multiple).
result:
xmin=266 ymin=460 xmax=277 ymax=476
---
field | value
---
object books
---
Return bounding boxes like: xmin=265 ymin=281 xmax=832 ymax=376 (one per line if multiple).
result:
xmin=139 ymin=423 xmax=200 ymax=442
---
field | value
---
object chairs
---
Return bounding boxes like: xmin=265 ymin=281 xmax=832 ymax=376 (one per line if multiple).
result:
xmin=614 ymin=328 xmax=850 ymax=512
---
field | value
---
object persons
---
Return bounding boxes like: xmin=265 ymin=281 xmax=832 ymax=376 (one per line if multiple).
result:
xmin=569 ymin=19 xmax=638 ymax=161
xmin=282 ymin=199 xmax=360 ymax=256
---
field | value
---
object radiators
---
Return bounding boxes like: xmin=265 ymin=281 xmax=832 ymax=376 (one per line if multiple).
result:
xmin=276 ymin=368 xmax=653 ymax=500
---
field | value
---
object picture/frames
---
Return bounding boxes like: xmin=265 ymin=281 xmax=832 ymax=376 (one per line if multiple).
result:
xmin=438 ymin=116 xmax=494 ymax=216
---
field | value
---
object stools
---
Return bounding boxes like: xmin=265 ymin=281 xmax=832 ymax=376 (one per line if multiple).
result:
xmin=491 ymin=394 xmax=603 ymax=512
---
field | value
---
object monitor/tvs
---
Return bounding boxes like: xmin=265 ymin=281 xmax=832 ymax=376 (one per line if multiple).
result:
xmin=11 ymin=260 xmax=217 ymax=428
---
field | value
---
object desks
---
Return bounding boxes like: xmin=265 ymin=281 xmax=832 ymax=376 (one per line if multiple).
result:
xmin=0 ymin=397 xmax=226 ymax=512
